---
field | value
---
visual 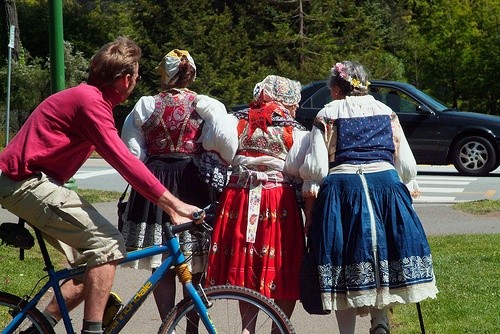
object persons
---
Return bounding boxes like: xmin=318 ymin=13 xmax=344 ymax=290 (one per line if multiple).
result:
xmin=385 ymin=91 xmax=402 ymax=112
xmin=205 ymin=75 xmax=315 ymax=334
xmin=120 ymin=49 xmax=238 ymax=334
xmin=299 ymin=60 xmax=437 ymax=334
xmin=0 ymin=38 xmax=205 ymax=334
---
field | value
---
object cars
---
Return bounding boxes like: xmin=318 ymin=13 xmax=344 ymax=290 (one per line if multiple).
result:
xmin=231 ymin=79 xmax=500 ymax=177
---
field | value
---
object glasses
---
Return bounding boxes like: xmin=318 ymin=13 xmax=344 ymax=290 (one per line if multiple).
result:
xmin=130 ymin=75 xmax=141 ymax=83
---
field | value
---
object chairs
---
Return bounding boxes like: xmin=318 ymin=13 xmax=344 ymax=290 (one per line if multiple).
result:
xmin=387 ymin=91 xmax=401 ymax=111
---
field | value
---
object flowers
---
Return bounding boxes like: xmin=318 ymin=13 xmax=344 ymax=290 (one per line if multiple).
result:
xmin=330 ymin=62 xmax=371 ymax=88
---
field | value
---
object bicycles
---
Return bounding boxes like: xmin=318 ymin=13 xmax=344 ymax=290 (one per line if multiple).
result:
xmin=0 ymin=200 xmax=297 ymax=334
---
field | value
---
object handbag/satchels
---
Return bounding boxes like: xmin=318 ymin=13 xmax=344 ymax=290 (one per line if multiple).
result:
xmin=118 ymin=184 xmax=130 ymax=233
xmin=300 ymin=226 xmax=329 ymax=315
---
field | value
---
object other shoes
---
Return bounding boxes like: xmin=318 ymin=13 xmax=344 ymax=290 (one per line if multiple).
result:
xmin=370 ymin=324 xmax=390 ymax=334
